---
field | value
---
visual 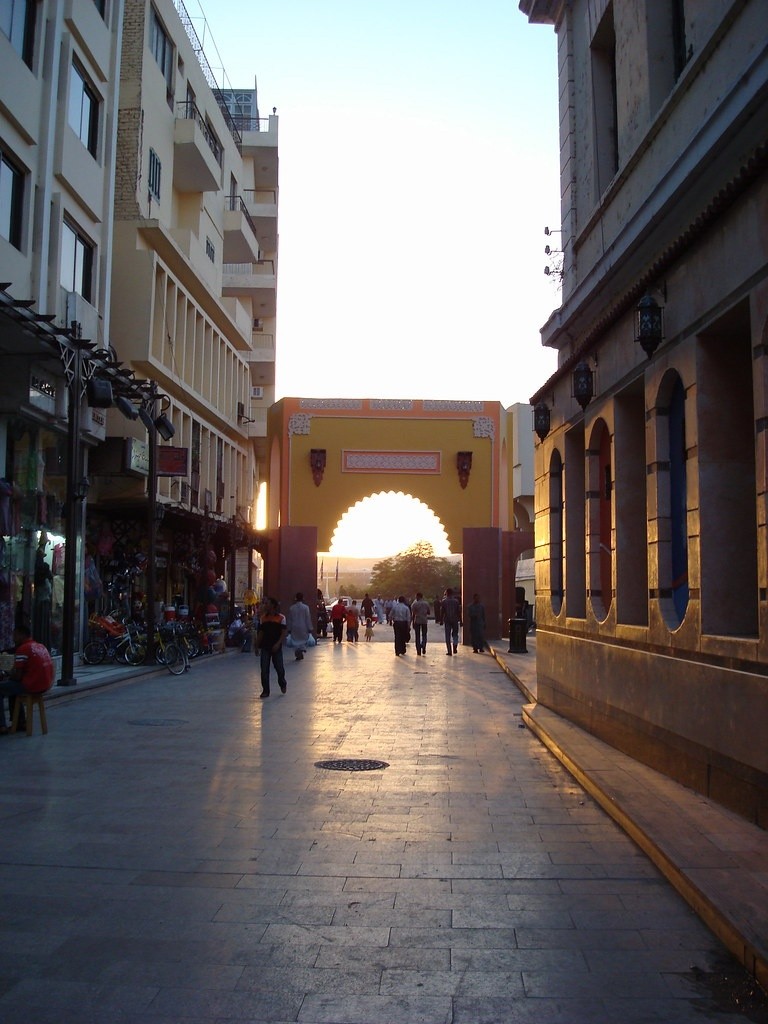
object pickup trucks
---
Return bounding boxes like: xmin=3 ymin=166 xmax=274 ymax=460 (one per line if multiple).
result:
xmin=325 ymin=598 xmax=361 ymax=616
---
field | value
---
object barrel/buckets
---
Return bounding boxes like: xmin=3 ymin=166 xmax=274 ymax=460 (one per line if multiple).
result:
xmin=164 ymin=607 xmax=176 ymax=622
xmin=179 ymin=605 xmax=189 ymax=619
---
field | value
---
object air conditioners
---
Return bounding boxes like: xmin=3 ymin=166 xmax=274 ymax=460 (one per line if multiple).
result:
xmin=252 ymin=385 xmax=264 ymax=399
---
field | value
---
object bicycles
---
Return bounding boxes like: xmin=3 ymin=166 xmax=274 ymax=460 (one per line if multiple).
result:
xmin=84 ymin=610 xmax=212 ymax=676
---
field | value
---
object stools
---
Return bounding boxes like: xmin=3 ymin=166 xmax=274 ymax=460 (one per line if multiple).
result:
xmin=11 ymin=693 xmax=48 ymax=737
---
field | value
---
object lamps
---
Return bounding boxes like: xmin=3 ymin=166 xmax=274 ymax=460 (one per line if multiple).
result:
xmin=84 ymin=355 xmax=114 ymax=407
xmin=209 ymin=516 xmax=218 ymax=536
xmin=132 ymin=383 xmax=157 ymax=432
xmin=77 ymin=477 xmax=90 ymax=501
xmin=111 ymin=367 xmax=140 ymax=420
xmin=454 ymin=450 xmax=473 ymax=489
xmin=141 ymin=394 xmax=176 ymax=441
xmin=154 ymin=501 xmax=168 ymax=521
xmin=544 ymin=266 xmax=563 ymax=276
xmin=310 ymin=447 xmax=328 ymax=486
xmin=545 ymin=227 xmax=566 ymax=236
xmin=534 ymin=390 xmax=555 ymax=444
xmin=545 ymin=244 xmax=565 ymax=256
xmin=571 ymin=347 xmax=599 ymax=410
xmin=633 ymin=278 xmax=670 ymax=360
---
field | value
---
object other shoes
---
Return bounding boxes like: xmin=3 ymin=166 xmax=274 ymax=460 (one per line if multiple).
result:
xmin=281 ymin=686 xmax=286 ymax=694
xmin=260 ymin=691 xmax=269 ymax=697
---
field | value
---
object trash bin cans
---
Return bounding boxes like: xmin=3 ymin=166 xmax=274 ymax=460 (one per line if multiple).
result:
xmin=508 ymin=617 xmax=527 ymax=653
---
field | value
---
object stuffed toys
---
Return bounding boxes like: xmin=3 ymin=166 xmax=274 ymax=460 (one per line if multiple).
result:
xmin=132 ymin=599 xmax=145 ymax=612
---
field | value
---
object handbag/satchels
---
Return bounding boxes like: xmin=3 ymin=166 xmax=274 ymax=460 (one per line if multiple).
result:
xmin=307 ymin=633 xmax=316 ymax=647
xmin=286 ymin=633 xmax=294 ymax=648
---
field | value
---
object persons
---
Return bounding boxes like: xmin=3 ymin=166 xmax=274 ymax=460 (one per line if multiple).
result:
xmin=0 ymin=567 xmax=18 ymax=651
xmin=52 ymin=566 xmax=65 ymax=612
xmin=255 ymin=598 xmax=287 ymax=697
xmin=360 ymin=593 xmax=414 ymax=625
xmin=467 ymin=593 xmax=486 ymax=653
xmin=0 ymin=624 xmax=54 ymax=735
xmin=228 ymin=613 xmax=248 ymax=646
xmin=439 ymin=588 xmax=463 ymax=655
xmin=389 ymin=596 xmax=411 ymax=656
xmin=287 ymin=593 xmax=313 ymax=661
xmin=329 ymin=599 xmax=375 ymax=643
xmin=411 ymin=592 xmax=430 ymax=655
xmin=434 ymin=595 xmax=442 ymax=623
xmin=36 ymin=563 xmax=53 ymax=636
